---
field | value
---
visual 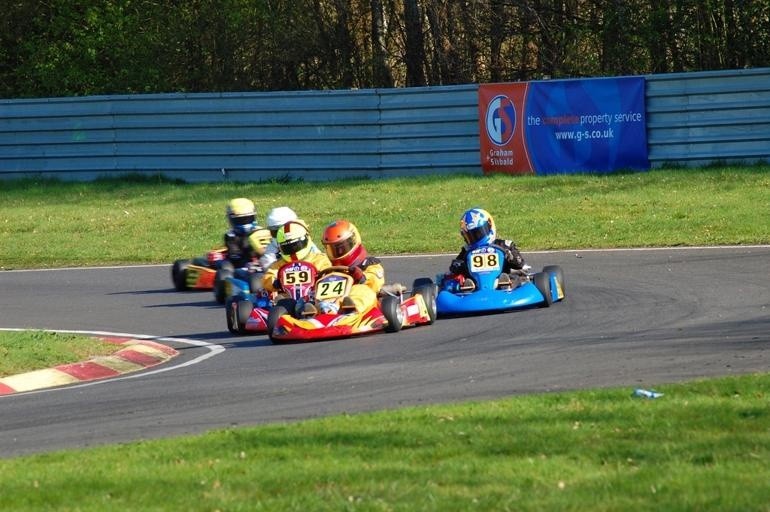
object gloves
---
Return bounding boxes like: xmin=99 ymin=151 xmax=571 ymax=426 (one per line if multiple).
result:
xmin=352 ymin=266 xmax=363 ymax=281
xmin=273 ymin=280 xmax=280 ymax=289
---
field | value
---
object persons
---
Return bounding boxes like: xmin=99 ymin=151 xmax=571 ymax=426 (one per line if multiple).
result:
xmin=444 ymin=208 xmax=530 ymax=293
xmin=206 ymin=197 xmax=385 ymax=318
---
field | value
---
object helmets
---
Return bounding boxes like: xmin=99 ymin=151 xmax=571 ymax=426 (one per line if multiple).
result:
xmin=459 ymin=208 xmax=496 ymax=252
xmin=321 ymin=221 xmax=363 ymax=267
xmin=226 ymin=198 xmax=257 ymax=235
xmin=276 ymin=222 xmax=312 ymax=263
xmin=267 ymin=207 xmax=298 ymax=247
